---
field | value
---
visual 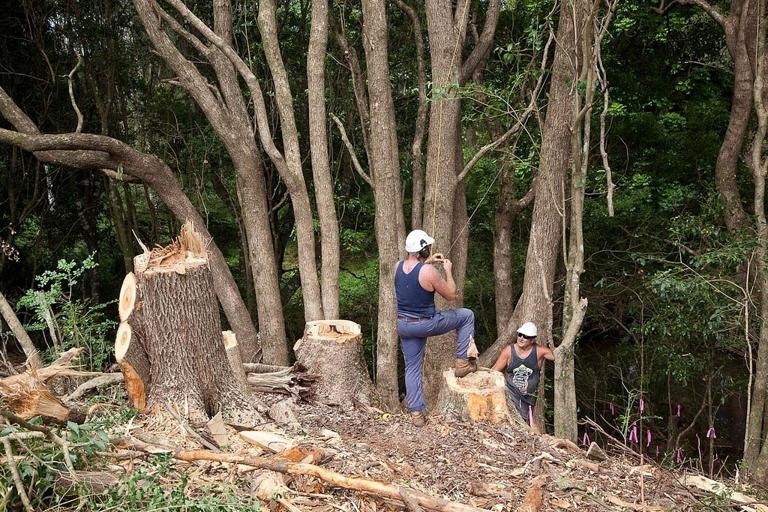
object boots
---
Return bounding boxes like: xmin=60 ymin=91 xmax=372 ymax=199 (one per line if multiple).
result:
xmin=454 ymin=360 xmax=478 ymax=377
xmin=412 ymin=410 xmax=424 ymax=426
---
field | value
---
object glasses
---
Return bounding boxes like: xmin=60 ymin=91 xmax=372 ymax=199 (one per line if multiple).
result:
xmin=517 ymin=332 xmax=533 ymax=339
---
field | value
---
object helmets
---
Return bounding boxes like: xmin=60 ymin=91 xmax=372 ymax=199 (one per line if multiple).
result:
xmin=517 ymin=321 xmax=538 ymax=337
xmin=405 ymin=229 xmax=435 ymax=252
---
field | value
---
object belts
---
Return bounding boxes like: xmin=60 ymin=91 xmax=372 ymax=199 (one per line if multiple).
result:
xmin=398 ymin=315 xmax=431 ymax=323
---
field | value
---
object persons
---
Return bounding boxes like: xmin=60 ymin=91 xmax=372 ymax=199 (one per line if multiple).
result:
xmin=392 ymin=228 xmax=477 ymax=427
xmin=490 ymin=321 xmax=555 ymax=428
